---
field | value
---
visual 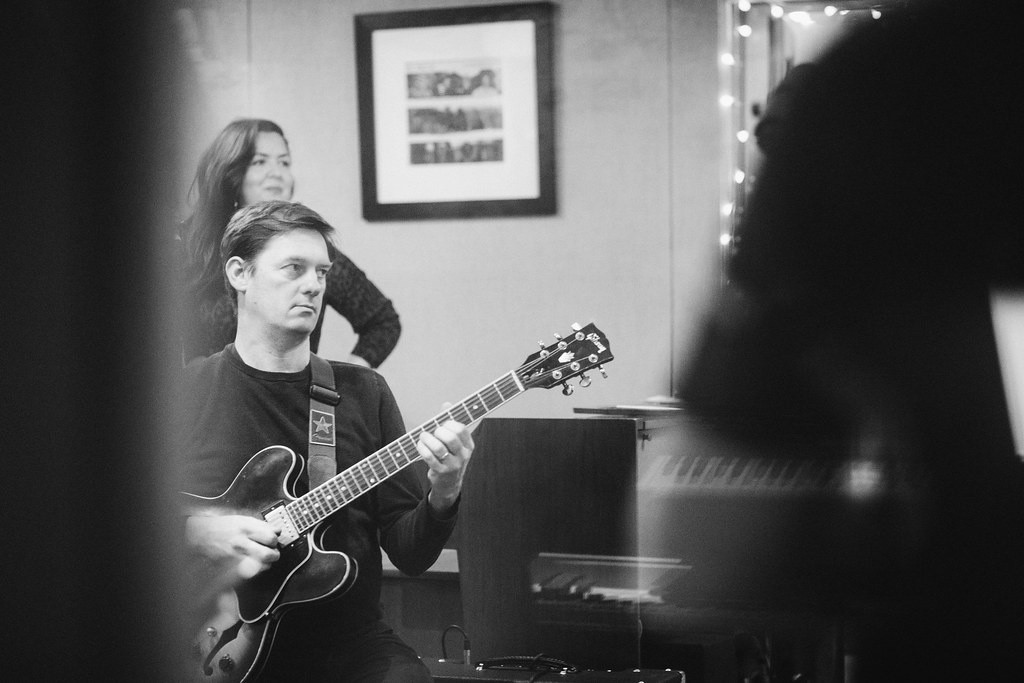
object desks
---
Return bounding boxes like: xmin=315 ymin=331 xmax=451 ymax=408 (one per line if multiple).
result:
xmin=379 ymin=546 xmax=464 ymax=658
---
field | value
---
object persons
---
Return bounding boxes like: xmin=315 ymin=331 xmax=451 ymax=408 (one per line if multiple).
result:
xmin=171 ymin=119 xmax=401 ymax=369
xmin=172 ymin=200 xmax=474 ymax=683
xmin=682 ymin=0 xmax=1024 ymax=683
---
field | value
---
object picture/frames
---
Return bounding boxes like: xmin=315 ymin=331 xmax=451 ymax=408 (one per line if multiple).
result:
xmin=353 ymin=2 xmax=555 ymax=224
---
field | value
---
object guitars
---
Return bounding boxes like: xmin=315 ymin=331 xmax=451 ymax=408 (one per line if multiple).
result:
xmin=176 ymin=318 xmax=616 ymax=683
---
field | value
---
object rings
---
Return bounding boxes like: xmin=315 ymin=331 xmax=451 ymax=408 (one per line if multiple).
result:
xmin=439 ymin=451 xmax=450 ymax=461
